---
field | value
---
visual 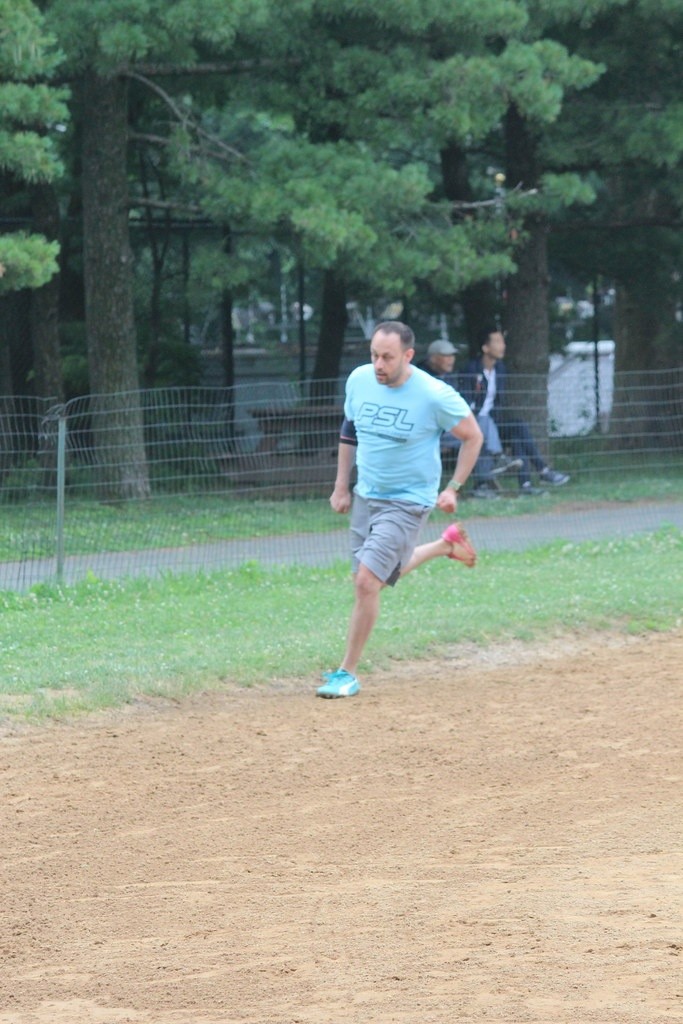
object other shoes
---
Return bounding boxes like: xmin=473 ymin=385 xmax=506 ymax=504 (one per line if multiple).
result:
xmin=539 ymin=468 xmax=568 ymax=486
xmin=518 ymin=485 xmax=547 ymax=497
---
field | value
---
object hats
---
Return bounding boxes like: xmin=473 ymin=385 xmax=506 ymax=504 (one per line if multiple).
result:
xmin=428 ymin=340 xmax=458 ymax=355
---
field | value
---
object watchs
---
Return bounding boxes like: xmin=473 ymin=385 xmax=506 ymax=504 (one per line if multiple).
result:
xmin=448 ymin=480 xmax=464 ymax=493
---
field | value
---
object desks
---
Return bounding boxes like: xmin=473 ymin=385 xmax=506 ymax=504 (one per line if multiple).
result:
xmin=249 ymin=407 xmax=344 ymax=459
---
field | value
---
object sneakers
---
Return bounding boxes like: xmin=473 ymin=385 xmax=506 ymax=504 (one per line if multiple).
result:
xmin=316 ymin=667 xmax=359 ymax=698
xmin=441 ymin=522 xmax=476 ymax=567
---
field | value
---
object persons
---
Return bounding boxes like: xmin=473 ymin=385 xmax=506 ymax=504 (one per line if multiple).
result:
xmin=416 ymin=325 xmax=570 ymax=499
xmin=315 ymin=322 xmax=484 ymax=698
